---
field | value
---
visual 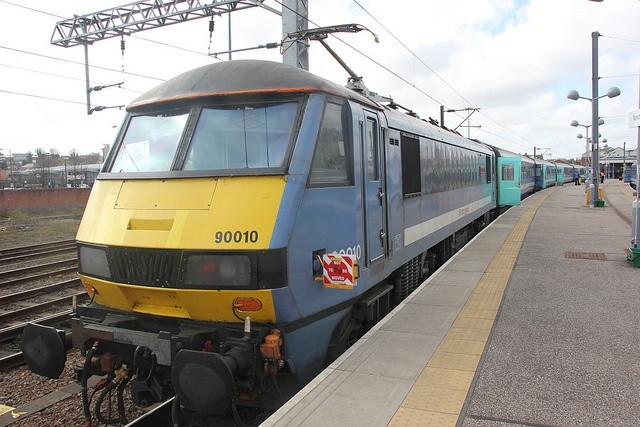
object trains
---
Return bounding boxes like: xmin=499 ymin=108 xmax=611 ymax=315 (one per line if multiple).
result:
xmin=20 ymin=60 xmax=591 ymax=422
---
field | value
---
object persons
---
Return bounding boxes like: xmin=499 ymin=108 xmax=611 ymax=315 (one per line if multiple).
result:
xmin=600 ymin=171 xmax=604 ymax=183
xmin=574 ymin=171 xmax=581 ymax=185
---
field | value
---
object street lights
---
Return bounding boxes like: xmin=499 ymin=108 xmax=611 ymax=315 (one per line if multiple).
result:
xmin=598 ymin=144 xmax=608 ymax=148
xmin=569 ymin=119 xmax=607 ymax=191
xmin=567 ymin=86 xmax=621 ymax=207
xmin=595 ymin=138 xmax=607 ymax=143
xmin=576 ymin=131 xmax=601 ymax=192
